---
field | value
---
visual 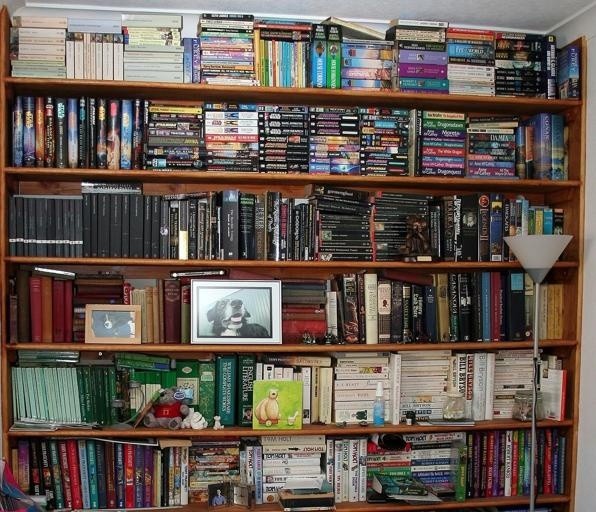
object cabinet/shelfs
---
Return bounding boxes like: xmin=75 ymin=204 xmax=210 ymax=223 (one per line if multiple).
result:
xmin=0 ymin=5 xmax=590 ymax=512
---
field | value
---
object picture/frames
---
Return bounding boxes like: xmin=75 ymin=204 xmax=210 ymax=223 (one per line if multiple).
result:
xmin=188 ymin=275 xmax=286 ymax=348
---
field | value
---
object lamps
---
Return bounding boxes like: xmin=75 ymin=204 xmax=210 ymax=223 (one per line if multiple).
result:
xmin=502 ymin=235 xmax=577 ymax=512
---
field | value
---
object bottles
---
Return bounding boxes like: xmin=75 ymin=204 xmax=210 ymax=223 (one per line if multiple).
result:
xmin=406 ymin=410 xmax=415 ymax=426
xmin=373 ymin=382 xmax=385 ymax=428
xmin=512 ymin=390 xmax=545 ymax=422
xmin=443 ymin=392 xmax=465 ymax=421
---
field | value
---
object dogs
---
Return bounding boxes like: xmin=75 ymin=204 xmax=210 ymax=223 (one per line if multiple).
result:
xmin=206 ymin=299 xmax=269 ymax=337
xmin=102 ymin=314 xmax=132 ymax=330
